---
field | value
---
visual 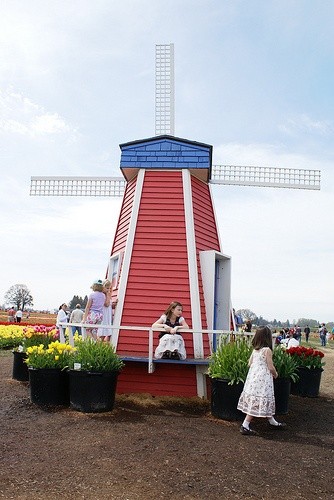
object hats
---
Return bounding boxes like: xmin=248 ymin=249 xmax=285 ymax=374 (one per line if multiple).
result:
xmin=95 ymin=280 xmax=102 ymax=285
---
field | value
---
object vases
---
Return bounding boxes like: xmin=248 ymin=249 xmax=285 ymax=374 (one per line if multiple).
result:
xmin=28 ymin=368 xmax=69 ymax=404
xmin=12 ymin=350 xmax=29 ymax=381
xmin=67 ymin=369 xmax=118 ymax=412
xmin=290 ymin=368 xmax=324 ymax=397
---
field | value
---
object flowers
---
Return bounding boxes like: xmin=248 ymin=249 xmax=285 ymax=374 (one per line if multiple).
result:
xmin=286 ymin=345 xmax=326 ymax=370
xmin=11 ymin=323 xmax=126 ymax=375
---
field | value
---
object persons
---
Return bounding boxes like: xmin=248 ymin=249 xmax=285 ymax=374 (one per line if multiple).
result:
xmin=7 ymin=306 xmax=29 ymax=323
xmin=237 ymin=326 xmax=287 ymax=436
xmin=56 ymin=279 xmax=119 ymax=345
xmin=152 ymin=301 xmax=189 ymax=360
xmin=232 ymin=308 xmax=334 ymax=350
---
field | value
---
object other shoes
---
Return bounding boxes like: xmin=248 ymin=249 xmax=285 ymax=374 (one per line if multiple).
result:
xmin=162 ymin=353 xmax=169 ymax=359
xmin=270 ymin=422 xmax=287 ymax=429
xmin=172 ymin=352 xmax=180 ymax=360
xmin=240 ymin=426 xmax=259 ymax=436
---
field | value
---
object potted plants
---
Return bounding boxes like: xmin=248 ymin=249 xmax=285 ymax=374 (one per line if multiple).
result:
xmin=272 ymin=344 xmax=300 ymax=415
xmin=205 ymin=332 xmax=253 ymax=420
